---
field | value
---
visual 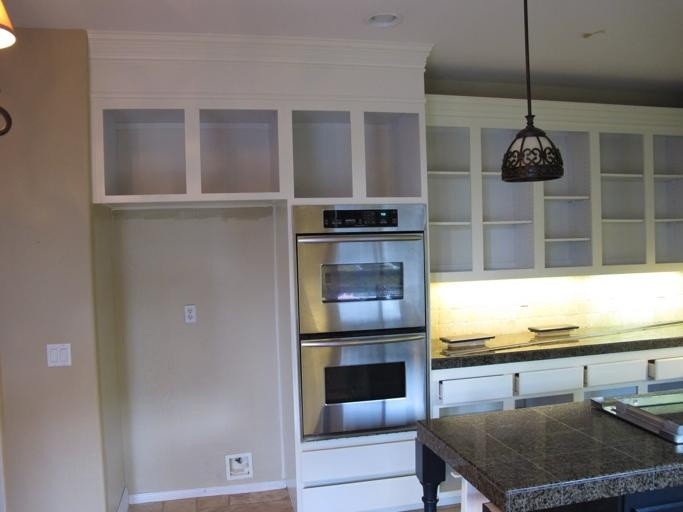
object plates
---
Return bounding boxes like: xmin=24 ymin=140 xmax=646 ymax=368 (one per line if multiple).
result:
xmin=528 ymin=325 xmax=579 ymax=337
xmin=439 ymin=334 xmax=495 ymax=348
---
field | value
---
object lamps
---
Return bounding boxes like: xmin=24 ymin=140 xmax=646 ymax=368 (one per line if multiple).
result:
xmin=501 ymin=0 xmax=565 ymax=184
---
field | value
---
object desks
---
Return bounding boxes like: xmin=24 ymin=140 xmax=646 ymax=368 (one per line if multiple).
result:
xmin=411 ymin=392 xmax=682 ymax=511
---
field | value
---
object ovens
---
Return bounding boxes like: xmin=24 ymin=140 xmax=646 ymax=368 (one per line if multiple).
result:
xmin=294 ymin=207 xmax=431 ymax=444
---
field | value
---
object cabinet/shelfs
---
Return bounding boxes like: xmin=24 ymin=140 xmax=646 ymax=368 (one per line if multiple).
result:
xmin=287 ymin=203 xmax=437 ymax=512
xmin=422 ymin=99 xmax=683 ymax=283
xmin=90 ymin=95 xmax=428 ymax=201
xmin=430 ymin=346 xmax=683 ymax=418
